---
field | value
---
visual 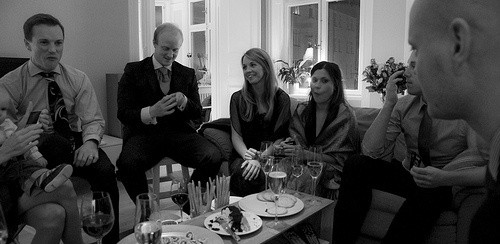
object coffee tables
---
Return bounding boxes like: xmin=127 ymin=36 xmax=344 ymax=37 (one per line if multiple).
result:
xmin=179 ymin=188 xmax=335 ymax=244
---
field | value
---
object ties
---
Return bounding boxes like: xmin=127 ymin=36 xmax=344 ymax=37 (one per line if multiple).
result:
xmin=41 ymin=72 xmax=76 ymax=154
xmin=159 ymin=66 xmax=169 ymax=82
xmin=418 ymin=104 xmax=432 ymax=167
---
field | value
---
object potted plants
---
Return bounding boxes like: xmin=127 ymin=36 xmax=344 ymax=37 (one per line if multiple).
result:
xmin=275 ymin=58 xmax=316 ymax=94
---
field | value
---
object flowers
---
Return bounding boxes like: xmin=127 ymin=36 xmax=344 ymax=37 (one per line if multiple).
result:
xmin=362 ymin=56 xmax=408 ymax=97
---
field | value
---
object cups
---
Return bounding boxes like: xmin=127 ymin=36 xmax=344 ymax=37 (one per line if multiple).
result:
xmin=134 ymin=193 xmax=161 ymax=244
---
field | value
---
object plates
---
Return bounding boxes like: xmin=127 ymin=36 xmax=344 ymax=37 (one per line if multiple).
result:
xmin=118 ymin=225 xmax=224 ymax=244
xmin=211 ymin=196 xmax=243 ymax=212
xmin=204 ymin=211 xmax=262 ymax=235
xmin=239 ymin=194 xmax=304 ymax=217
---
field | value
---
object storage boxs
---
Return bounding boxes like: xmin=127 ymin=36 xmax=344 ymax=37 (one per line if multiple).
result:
xmin=106 ymin=73 xmax=125 ymax=137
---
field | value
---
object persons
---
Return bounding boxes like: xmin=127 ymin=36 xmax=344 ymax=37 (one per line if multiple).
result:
xmin=229 ymin=48 xmax=292 ymax=197
xmin=115 ymin=23 xmax=222 ymax=219
xmin=270 ymin=62 xmax=357 ymax=239
xmin=332 ymin=0 xmax=500 ymax=244
xmin=0 ymin=14 xmax=120 ymax=244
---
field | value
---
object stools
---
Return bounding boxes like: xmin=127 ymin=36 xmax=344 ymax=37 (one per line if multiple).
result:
xmin=146 ymin=156 xmax=189 ymax=211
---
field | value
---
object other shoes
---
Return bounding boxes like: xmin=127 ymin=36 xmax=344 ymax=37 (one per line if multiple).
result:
xmin=41 ymin=164 xmax=73 ymax=193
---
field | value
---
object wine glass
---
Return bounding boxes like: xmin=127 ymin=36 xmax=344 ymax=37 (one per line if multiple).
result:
xmin=257 ymin=141 xmax=275 ymax=201
xmin=306 ymin=144 xmax=324 ymax=206
xmin=170 ymin=176 xmax=189 ymax=221
xmin=266 ymin=157 xmax=289 ymax=229
xmin=291 ymin=147 xmax=304 ymax=198
xmin=81 ymin=191 xmax=114 ymax=244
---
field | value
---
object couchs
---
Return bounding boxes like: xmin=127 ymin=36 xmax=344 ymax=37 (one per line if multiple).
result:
xmin=198 ymin=102 xmax=407 ymax=244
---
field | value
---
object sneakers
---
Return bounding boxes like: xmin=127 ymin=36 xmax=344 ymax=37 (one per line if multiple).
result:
xmin=140 ymin=207 xmax=151 ymax=223
xmin=172 ymin=193 xmax=190 ymax=215
